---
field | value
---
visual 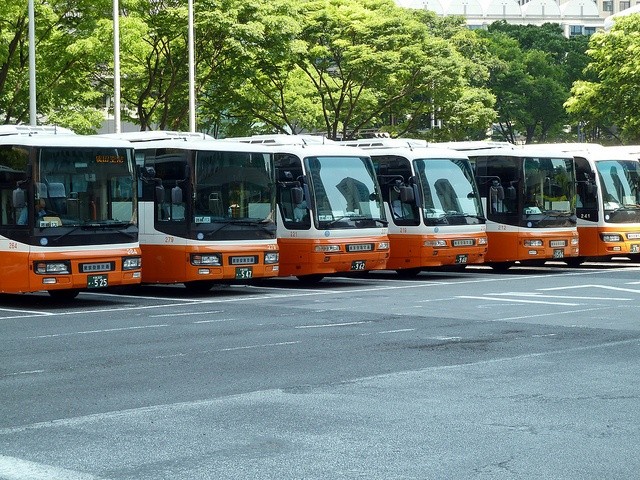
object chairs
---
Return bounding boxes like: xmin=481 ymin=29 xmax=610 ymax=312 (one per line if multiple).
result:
xmin=35 ymin=183 xmax=57 ymax=218
xmin=47 ymin=182 xmax=70 ymax=218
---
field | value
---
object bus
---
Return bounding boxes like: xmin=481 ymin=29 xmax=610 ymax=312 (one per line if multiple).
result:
xmin=97 ymin=129 xmax=279 ymax=298
xmin=0 ymin=124 xmax=145 ymax=308
xmin=221 ymin=135 xmax=390 ymax=286
xmin=522 ymin=144 xmax=640 ymax=266
xmin=333 ymin=137 xmax=489 ymax=278
xmin=427 ymin=139 xmax=581 ymax=272
